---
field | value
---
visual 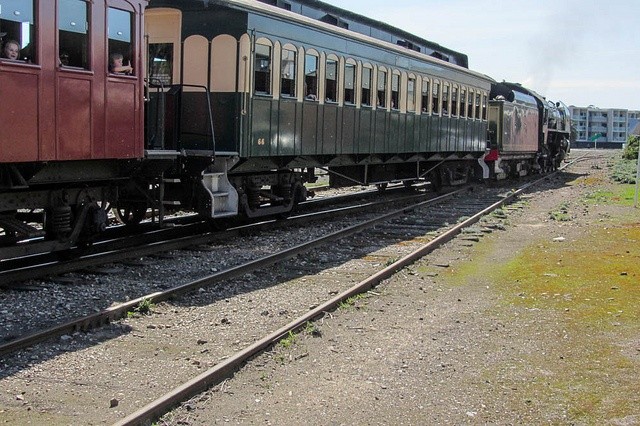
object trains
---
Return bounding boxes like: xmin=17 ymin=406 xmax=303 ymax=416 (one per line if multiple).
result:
xmin=1 ymin=0 xmax=572 ymax=261
